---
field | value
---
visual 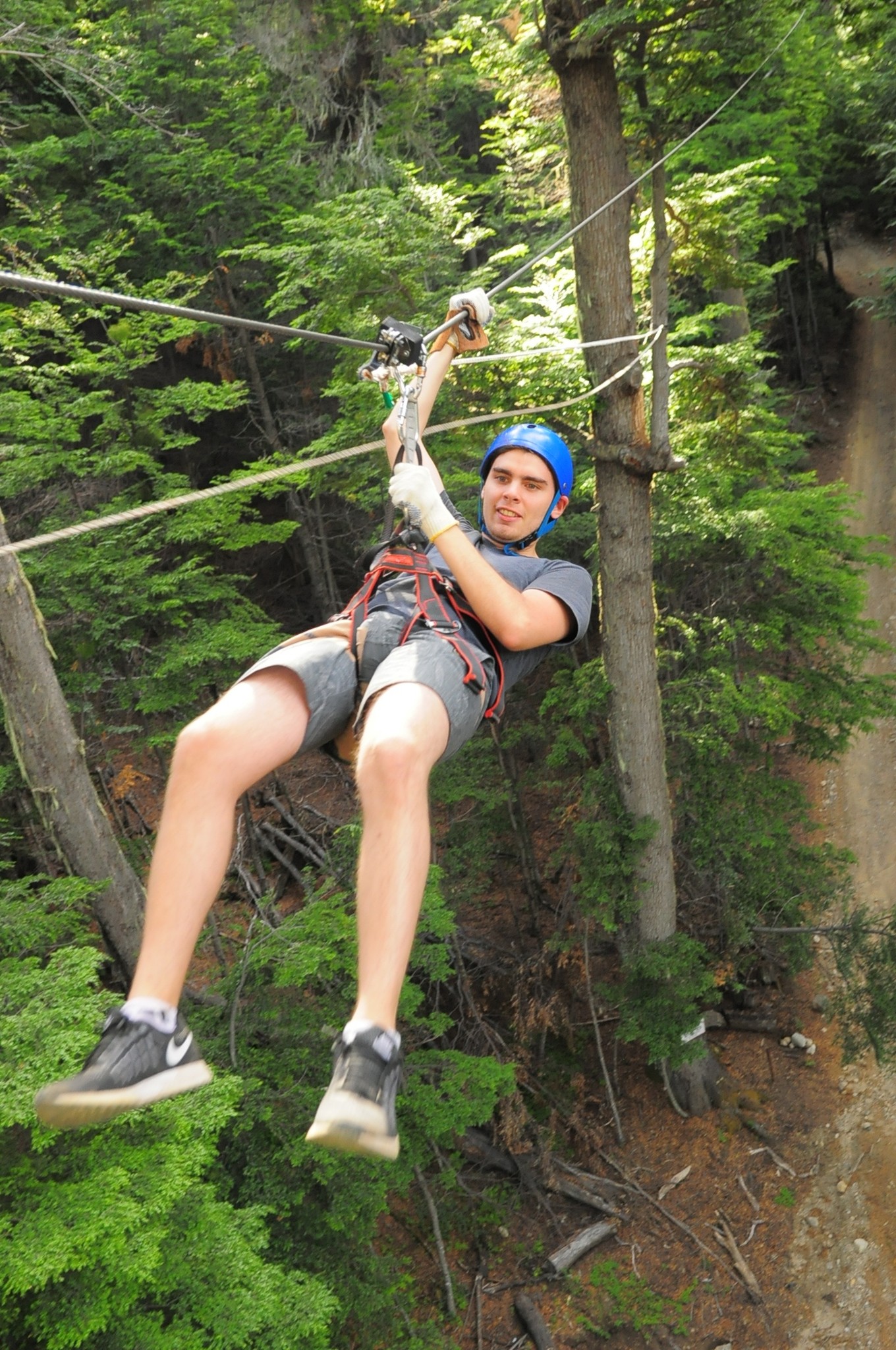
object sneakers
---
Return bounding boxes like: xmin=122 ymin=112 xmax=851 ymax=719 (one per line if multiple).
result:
xmin=305 ymin=1026 xmax=402 ymax=1161
xmin=34 ymin=1009 xmax=213 ymax=1126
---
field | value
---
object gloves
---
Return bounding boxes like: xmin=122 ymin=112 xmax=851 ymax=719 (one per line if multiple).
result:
xmin=388 ymin=462 xmax=459 ymax=541
xmin=446 ymin=287 xmax=489 ymax=352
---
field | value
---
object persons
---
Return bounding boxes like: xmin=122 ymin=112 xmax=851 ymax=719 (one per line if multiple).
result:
xmin=32 ymin=286 xmax=593 ymax=1160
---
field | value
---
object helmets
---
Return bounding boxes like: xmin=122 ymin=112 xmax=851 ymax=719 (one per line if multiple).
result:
xmin=480 ymin=423 xmax=574 ymax=497
